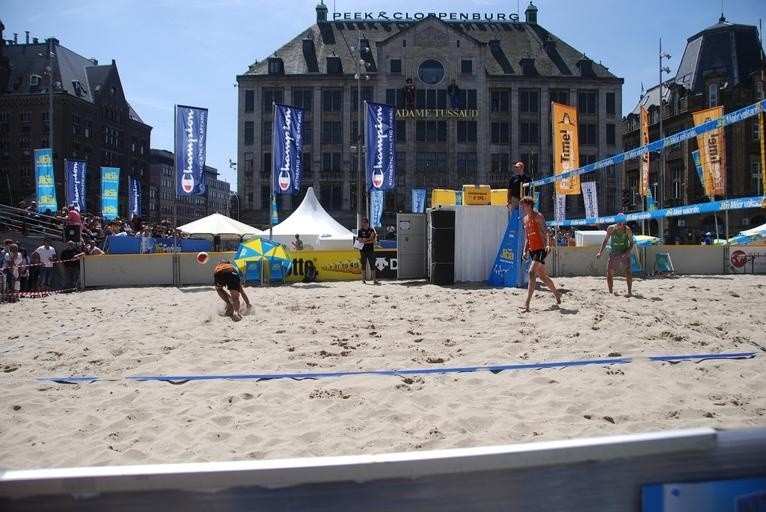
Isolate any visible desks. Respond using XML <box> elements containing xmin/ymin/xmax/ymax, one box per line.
<box><xmin>738</xmin><ymin>253</ymin><xmax>766</xmax><ymax>273</ymax></box>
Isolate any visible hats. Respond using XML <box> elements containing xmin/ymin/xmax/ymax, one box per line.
<box><xmin>515</xmin><ymin>162</ymin><xmax>526</xmax><ymax>171</ymax></box>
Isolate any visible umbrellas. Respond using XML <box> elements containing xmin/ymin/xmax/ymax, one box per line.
<box><xmin>176</xmin><ymin>213</ymin><xmax>262</xmax><ymax>251</ymax></box>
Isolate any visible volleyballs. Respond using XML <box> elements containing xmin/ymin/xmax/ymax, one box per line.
<box><xmin>197</xmin><ymin>251</ymin><xmax>208</xmax><ymax>264</ymax></box>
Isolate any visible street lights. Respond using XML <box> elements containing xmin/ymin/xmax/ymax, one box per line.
<box><xmin>346</xmin><ymin>32</ymin><xmax>370</xmax><ymax>239</ymax></box>
<box><xmin>657</xmin><ymin>36</ymin><xmax>672</xmax><ymax>246</ymax></box>
<box><xmin>37</xmin><ymin>38</ymin><xmax>63</xmax><ymax>152</ymax></box>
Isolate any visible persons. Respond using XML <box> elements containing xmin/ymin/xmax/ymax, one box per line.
<box><xmin>1</xmin><ymin>239</ymin><xmax>56</xmax><ymax>303</ymax></box>
<box><xmin>358</xmin><ymin>218</ymin><xmax>381</xmax><ymax>285</ymax></box>
<box><xmin>597</xmin><ymin>213</ymin><xmax>633</xmax><ymax>297</ymax></box>
<box><xmin>61</xmin><ymin>240</ymin><xmax>105</xmax><ymax>293</ymax></box>
<box><xmin>214</xmin><ymin>259</ymin><xmax>251</xmax><ymax>321</ymax></box>
<box><xmin>507</xmin><ymin>162</ymin><xmax>532</xmax><ymax>211</ymax></box>
<box><xmin>292</xmin><ymin>234</ymin><xmax>303</xmax><ymax>250</ymax></box>
<box><xmin>0</xmin><ymin>201</ymin><xmax>191</xmax><ymax>242</ymax></box>
<box><xmin>518</xmin><ymin>197</ymin><xmax>562</xmax><ymax>310</ymax></box>
<box><xmin>386</xmin><ymin>224</ymin><xmax>395</xmax><ymax>239</ymax></box>
